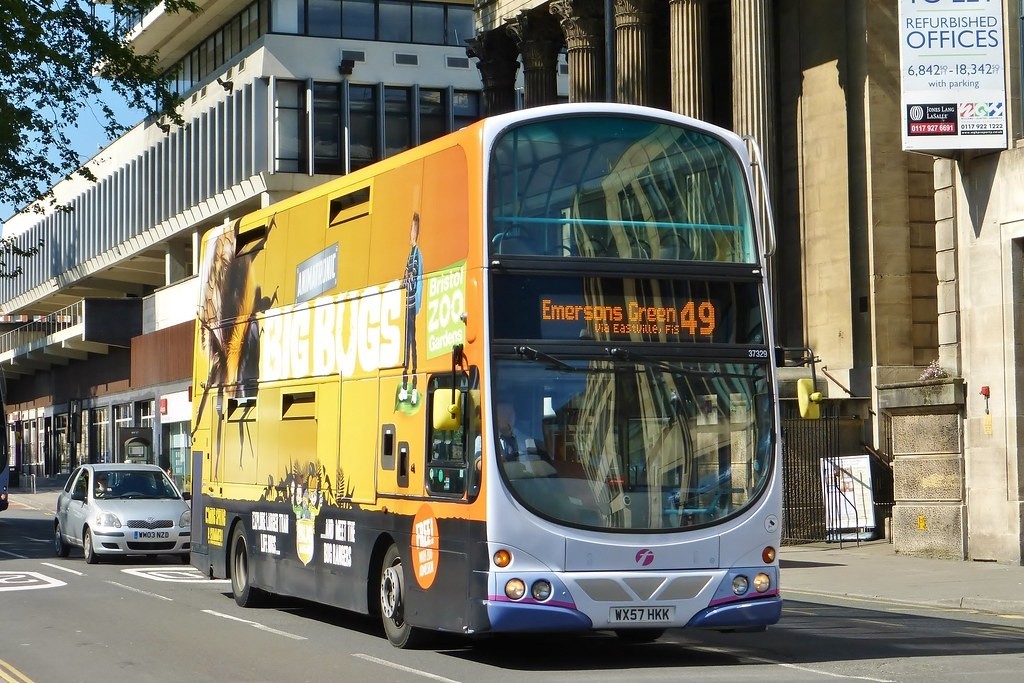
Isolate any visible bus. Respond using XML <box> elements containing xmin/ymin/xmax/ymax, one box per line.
<box><xmin>185</xmin><ymin>98</ymin><xmax>823</xmax><ymax>650</ymax></box>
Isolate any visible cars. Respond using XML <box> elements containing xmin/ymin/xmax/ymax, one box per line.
<box><xmin>52</xmin><ymin>463</ymin><xmax>193</xmax><ymax>564</ymax></box>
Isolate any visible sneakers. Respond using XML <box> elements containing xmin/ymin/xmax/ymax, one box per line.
<box><xmin>398</xmin><ymin>388</ymin><xmax>408</xmax><ymax>401</ymax></box>
<box><xmin>411</xmin><ymin>389</ymin><xmax>418</xmax><ymax>404</ymax></box>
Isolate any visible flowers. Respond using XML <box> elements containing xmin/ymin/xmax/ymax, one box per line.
<box><xmin>917</xmin><ymin>358</ymin><xmax>952</xmax><ymax>381</ymax></box>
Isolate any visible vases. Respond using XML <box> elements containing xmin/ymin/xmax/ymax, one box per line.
<box><xmin>875</xmin><ymin>377</ymin><xmax>964</xmax><ymax>409</ymax></box>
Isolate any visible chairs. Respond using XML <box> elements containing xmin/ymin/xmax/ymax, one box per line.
<box><xmin>659</xmin><ymin>234</ymin><xmax>695</xmax><ymax>260</ymax></box>
<box><xmin>492</xmin><ymin>231</ymin><xmax>540</xmax><ymax>255</ymax></box>
<box><xmin>548</xmin><ymin>246</ymin><xmax>577</xmax><ymax>255</ymax></box>
<box><xmin>605</xmin><ymin>228</ymin><xmax>653</xmax><ymax>258</ymax></box>
<box><xmin>571</xmin><ymin>238</ymin><xmax>608</xmax><ymax>257</ymax></box>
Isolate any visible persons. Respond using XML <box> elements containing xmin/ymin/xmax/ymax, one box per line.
<box><xmin>163</xmin><ymin>469</ymin><xmax>175</xmax><ymax>486</ymax></box>
<box><xmin>95</xmin><ymin>474</ymin><xmax>112</xmax><ymax>497</ymax></box>
<box><xmin>398</xmin><ymin>213</ymin><xmax>419</xmax><ymax>405</ymax></box>
<box><xmin>475</xmin><ymin>403</ymin><xmax>531</xmax><ymax>471</ymax></box>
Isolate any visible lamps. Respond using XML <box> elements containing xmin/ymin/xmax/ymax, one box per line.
<box><xmin>338</xmin><ymin>58</ymin><xmax>355</xmax><ymax>75</ymax></box>
<box><xmin>217</xmin><ymin>78</ymin><xmax>233</xmax><ymax>91</ymax></box>
